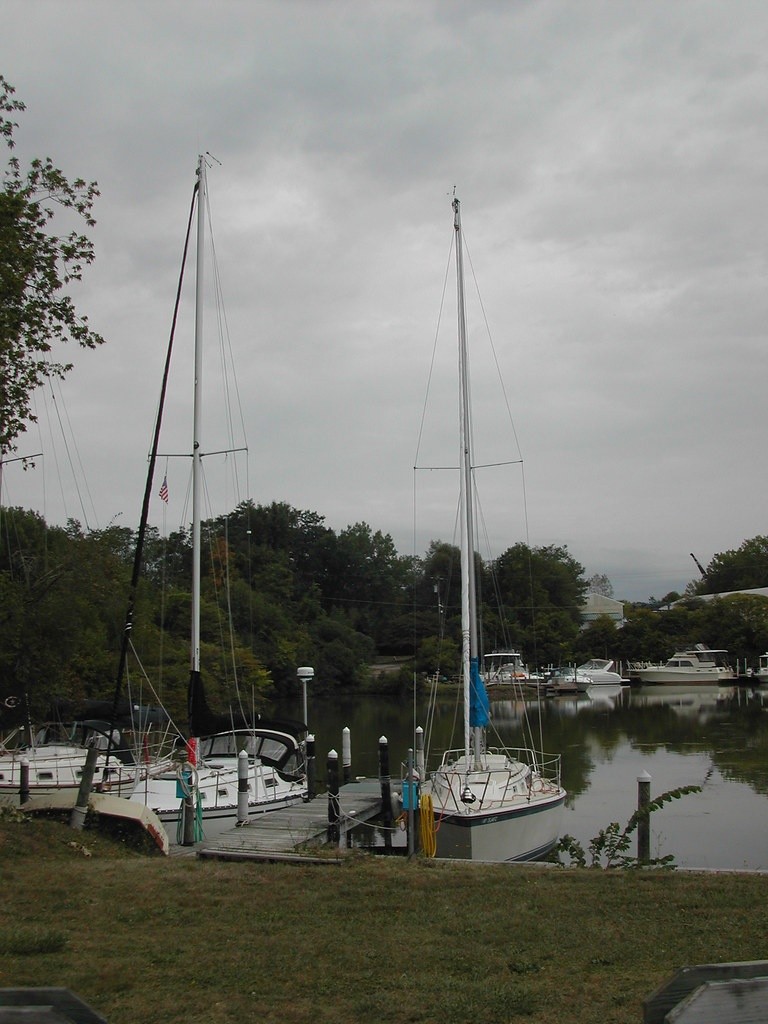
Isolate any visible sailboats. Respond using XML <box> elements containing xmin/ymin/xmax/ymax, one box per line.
<box><xmin>403</xmin><ymin>186</ymin><xmax>571</xmax><ymax>863</ymax></box>
<box><xmin>0</xmin><ymin>153</ymin><xmax>318</xmax><ymax>845</ymax></box>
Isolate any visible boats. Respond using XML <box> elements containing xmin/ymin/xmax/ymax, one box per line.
<box><xmin>479</xmin><ymin>642</ymin><xmax>768</xmax><ymax>692</ymax></box>
